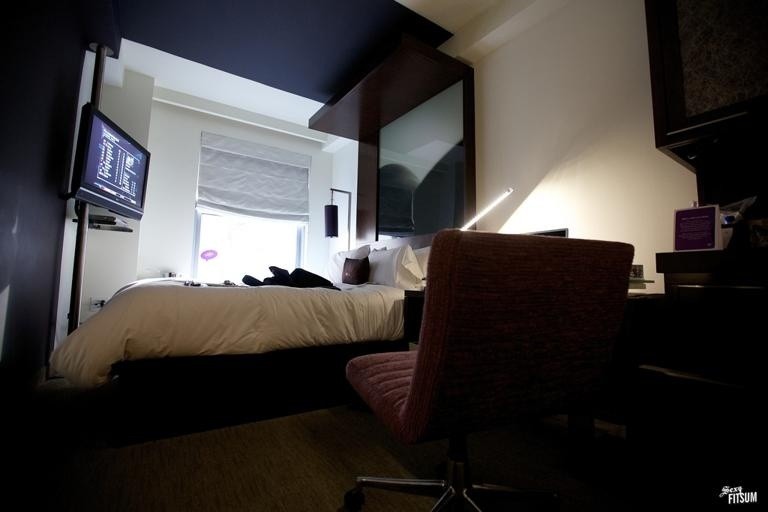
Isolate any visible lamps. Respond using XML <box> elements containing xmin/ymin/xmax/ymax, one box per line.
<box><xmin>323</xmin><ymin>188</ymin><xmax>351</xmax><ymax>252</ymax></box>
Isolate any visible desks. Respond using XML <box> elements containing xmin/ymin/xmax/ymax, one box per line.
<box><xmin>403</xmin><ymin>291</ymin><xmax>665</xmax><ymax>484</ymax></box>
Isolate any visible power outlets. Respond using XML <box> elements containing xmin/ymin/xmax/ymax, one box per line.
<box><xmin>90</xmin><ymin>297</ymin><xmax>107</xmax><ymax>312</ymax></box>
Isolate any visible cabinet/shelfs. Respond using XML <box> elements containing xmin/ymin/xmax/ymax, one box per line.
<box><xmin>638</xmin><ymin>0</ymin><xmax>767</xmax><ymax>512</ymax></box>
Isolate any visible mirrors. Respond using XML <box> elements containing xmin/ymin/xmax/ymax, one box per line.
<box><xmin>356</xmin><ymin>70</ymin><xmax>477</xmax><ymax>246</ymax></box>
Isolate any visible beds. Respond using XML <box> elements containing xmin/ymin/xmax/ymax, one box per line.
<box><xmin>46</xmin><ymin>242</ymin><xmax>432</xmax><ymax>444</ymax></box>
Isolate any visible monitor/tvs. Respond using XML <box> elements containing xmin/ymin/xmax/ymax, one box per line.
<box><xmin>72</xmin><ymin>101</ymin><xmax>151</xmax><ymax>222</ymax></box>
<box><xmin>523</xmin><ymin>228</ymin><xmax>568</xmax><ymax>238</ymax></box>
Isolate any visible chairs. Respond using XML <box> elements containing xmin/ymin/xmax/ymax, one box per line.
<box><xmin>344</xmin><ymin>229</ymin><xmax>634</xmax><ymax>511</ymax></box>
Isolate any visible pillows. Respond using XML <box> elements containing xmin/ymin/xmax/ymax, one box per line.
<box><xmin>342</xmin><ymin>256</ymin><xmax>369</xmax><ymax>285</ymax></box>
<box><xmin>367</xmin><ymin>245</ymin><xmax>424</xmax><ymax>289</ymax></box>
<box><xmin>322</xmin><ymin>246</ymin><xmax>369</xmax><ymax>284</ymax></box>
